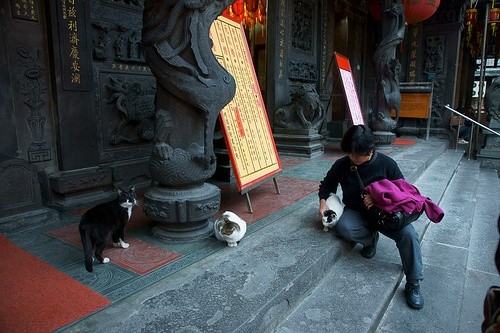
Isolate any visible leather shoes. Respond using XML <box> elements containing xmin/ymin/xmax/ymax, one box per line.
<box><xmin>405</xmin><ymin>282</ymin><xmax>424</xmax><ymax>308</ymax></box>
<box><xmin>361</xmin><ymin>233</ymin><xmax>380</xmax><ymax>258</ymax></box>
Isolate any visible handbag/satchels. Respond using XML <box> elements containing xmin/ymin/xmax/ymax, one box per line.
<box><xmin>350</xmin><ymin>160</ymin><xmax>424</xmax><ymax>230</ymax></box>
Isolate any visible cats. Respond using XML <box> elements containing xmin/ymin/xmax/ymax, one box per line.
<box><xmin>214</xmin><ymin>211</ymin><xmax>247</xmax><ymax>247</ymax></box>
<box><xmin>320</xmin><ymin>193</ymin><xmax>345</xmax><ymax>231</ymax></box>
<box><xmin>78</xmin><ymin>184</ymin><xmax>144</xmax><ymax>273</ymax></box>
